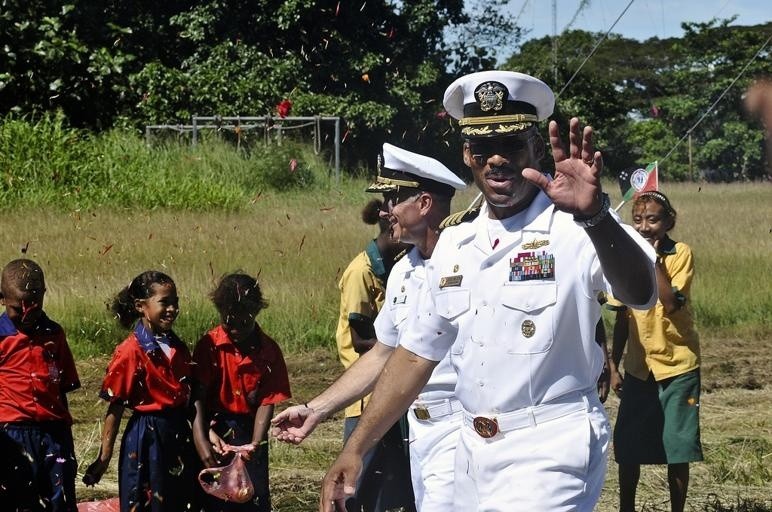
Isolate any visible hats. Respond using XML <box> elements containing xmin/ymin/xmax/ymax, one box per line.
<box><xmin>441</xmin><ymin>70</ymin><xmax>556</xmax><ymax>140</ymax></box>
<box><xmin>365</xmin><ymin>143</ymin><xmax>467</xmax><ymax>196</ymax></box>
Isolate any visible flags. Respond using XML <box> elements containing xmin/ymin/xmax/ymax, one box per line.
<box><xmin>615</xmin><ymin>160</ymin><xmax>657</xmax><ymax>200</ymax></box>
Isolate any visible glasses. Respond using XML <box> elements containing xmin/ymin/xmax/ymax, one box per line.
<box><xmin>466</xmin><ymin>134</ymin><xmax>536</xmax><ymax>157</ymax></box>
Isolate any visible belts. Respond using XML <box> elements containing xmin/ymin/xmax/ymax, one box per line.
<box><xmin>463</xmin><ymin>387</ymin><xmax>601</xmax><ymax>438</ymax></box>
<box><xmin>407</xmin><ymin>394</ymin><xmax>463</xmax><ymax>422</ymax></box>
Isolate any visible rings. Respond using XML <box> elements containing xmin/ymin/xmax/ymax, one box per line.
<box><xmin>331</xmin><ymin>500</ymin><xmax>336</xmax><ymax>504</ymax></box>
<box><xmin>579</xmin><ymin>153</ymin><xmax>595</xmax><ymax>164</ymax></box>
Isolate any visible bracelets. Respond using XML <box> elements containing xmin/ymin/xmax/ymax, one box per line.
<box><xmin>571</xmin><ymin>191</ymin><xmax>611</xmax><ymax>230</ymax></box>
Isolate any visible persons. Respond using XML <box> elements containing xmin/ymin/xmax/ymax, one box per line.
<box><xmin>319</xmin><ymin>71</ymin><xmax>656</xmax><ymax>511</ymax></box>
<box><xmin>608</xmin><ymin>186</ymin><xmax>705</xmax><ymax>511</ymax></box>
<box><xmin>79</xmin><ymin>271</ymin><xmax>231</xmax><ymax>511</ymax></box>
<box><xmin>187</xmin><ymin>269</ymin><xmax>292</xmax><ymax>512</ymax></box>
<box><xmin>592</xmin><ymin>289</ymin><xmax>611</xmax><ymax>404</ymax></box>
<box><xmin>330</xmin><ymin>196</ymin><xmax>416</xmax><ymax>511</ymax></box>
<box><xmin>2</xmin><ymin>258</ymin><xmax>85</xmax><ymax>511</ymax></box>
<box><xmin>267</xmin><ymin>141</ymin><xmax>469</xmax><ymax>512</ymax></box>
